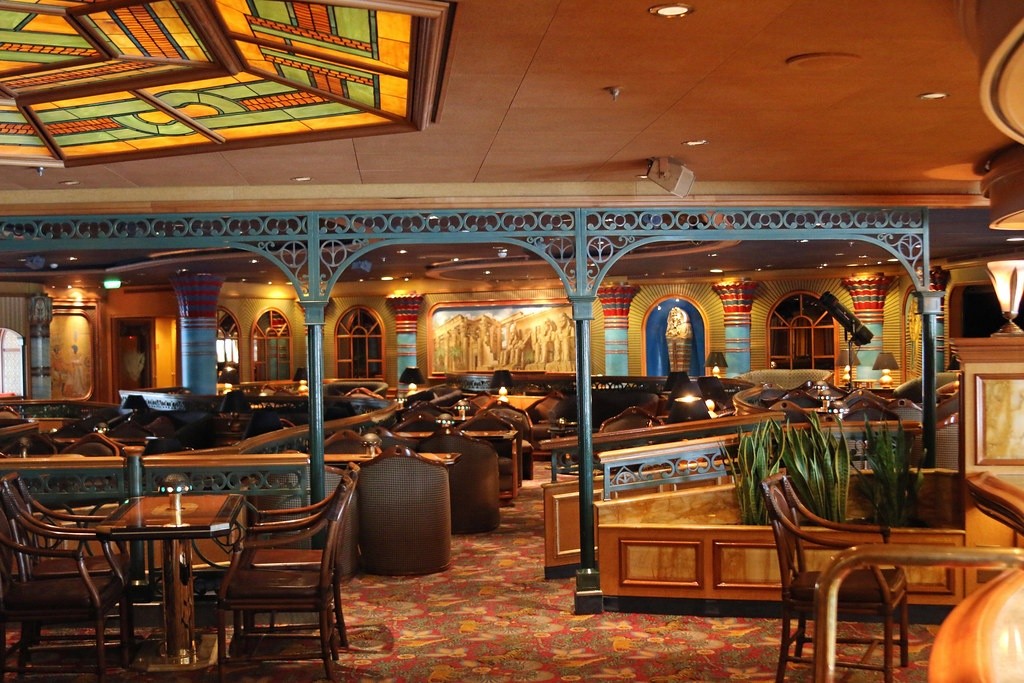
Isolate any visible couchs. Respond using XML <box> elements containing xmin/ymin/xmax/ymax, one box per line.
<box><xmin>0</xmin><ymin>369</ymin><xmax>962</xmax><ymax>576</ymax></box>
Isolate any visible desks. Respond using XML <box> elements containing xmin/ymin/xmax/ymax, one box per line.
<box><xmin>393</xmin><ymin>431</ymin><xmax>519</xmax><ymax>498</ymax></box>
<box><xmin>95</xmin><ymin>494</ymin><xmax>244</xmax><ymax>669</ymax></box>
<box><xmin>324</xmin><ymin>453</ymin><xmax>464</xmax><ymax>474</ymax></box>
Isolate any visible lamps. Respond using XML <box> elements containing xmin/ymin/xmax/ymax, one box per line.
<box><xmin>104</xmin><ymin>273</ymin><xmax>122</xmax><ymax>289</ymax></box>
<box><xmin>836</xmin><ymin>349</ymin><xmax>861</xmax><ymax>383</ymax></box>
<box><xmin>647</xmin><ymin>158</ymin><xmax>696</xmax><ymax>198</ymax></box>
<box><xmin>703</xmin><ymin>352</ymin><xmax>728</xmax><ymax>378</ymax></box>
<box><xmin>25</xmin><ymin>256</ymin><xmax>45</xmax><ymax>269</ymax></box>
<box><xmin>293</xmin><ymin>368</ymin><xmax>309</xmax><ymax>396</ymax></box>
<box><xmin>667</xmin><ymin>397</ymin><xmax>711</xmax><ymax>424</ymax></box>
<box><xmin>399</xmin><ymin>367</ymin><xmax>425</xmax><ymax>395</ymax></box>
<box><xmin>664</xmin><ymin>383</ymin><xmax>709</xmax><ymax>411</ymax></box>
<box><xmin>122</xmin><ymin>395</ymin><xmax>149</xmax><ymax>421</ymax></box>
<box><xmin>872</xmin><ymin>352</ymin><xmax>899</xmax><ymax>388</ymax></box>
<box><xmin>491</xmin><ymin>370</ymin><xmax>513</xmax><ymax>404</ymax></box>
<box><xmin>663</xmin><ymin>372</ymin><xmax>691</xmax><ymax>392</ymax></box>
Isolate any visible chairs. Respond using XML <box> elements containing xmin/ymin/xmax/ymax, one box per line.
<box><xmin>0</xmin><ymin>462</ymin><xmax>361</xmax><ymax>683</ymax></box>
<box><xmin>761</xmin><ymin>473</ymin><xmax>908</xmax><ymax>683</ymax></box>
